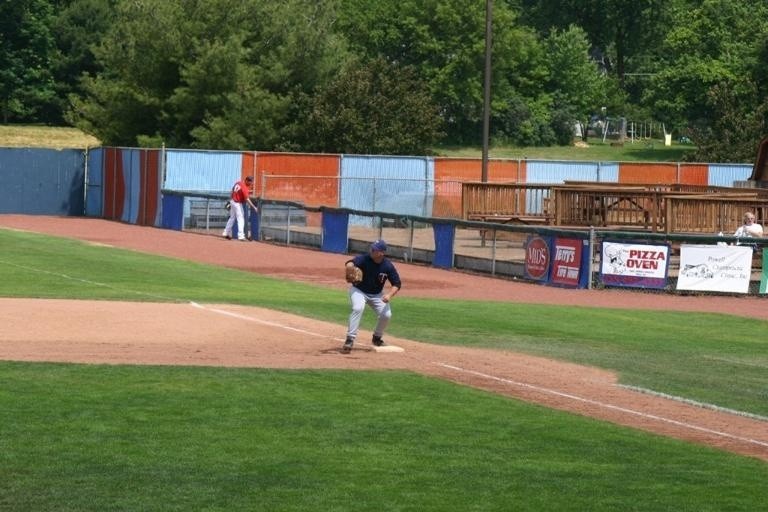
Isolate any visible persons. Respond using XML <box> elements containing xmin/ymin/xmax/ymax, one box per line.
<box><xmin>734</xmin><ymin>212</ymin><xmax>763</xmax><ymax>253</ymax></box>
<box><xmin>343</xmin><ymin>240</ymin><xmax>401</xmax><ymax>351</ymax></box>
<box><xmin>223</xmin><ymin>176</ymin><xmax>257</xmax><ymax>241</ymax></box>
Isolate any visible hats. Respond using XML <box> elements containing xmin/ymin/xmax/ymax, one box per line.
<box><xmin>246</xmin><ymin>176</ymin><xmax>254</xmax><ymax>183</ymax></box>
<box><xmin>370</xmin><ymin>239</ymin><xmax>387</xmax><ymax>251</ymax></box>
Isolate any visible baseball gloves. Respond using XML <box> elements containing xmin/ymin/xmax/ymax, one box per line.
<box><xmin>346</xmin><ymin>267</ymin><xmax>363</xmax><ymax>283</ymax></box>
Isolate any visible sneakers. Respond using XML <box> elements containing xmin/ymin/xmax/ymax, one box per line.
<box><xmin>372</xmin><ymin>339</ymin><xmax>385</xmax><ymax>346</ymax></box>
<box><xmin>224</xmin><ymin>235</ymin><xmax>231</xmax><ymax>240</ymax></box>
<box><xmin>342</xmin><ymin>339</ymin><xmax>352</xmax><ymax>350</ymax></box>
<box><xmin>239</xmin><ymin>238</ymin><xmax>250</xmax><ymax>242</ymax></box>
